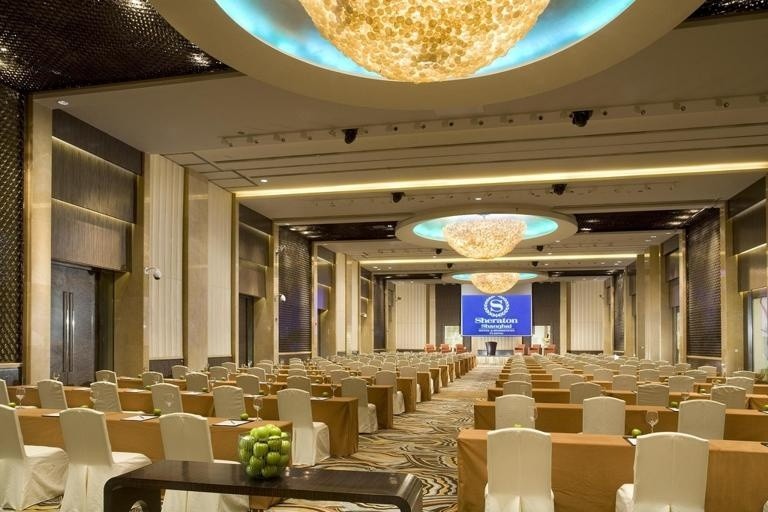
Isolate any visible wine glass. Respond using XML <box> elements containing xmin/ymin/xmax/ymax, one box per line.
<box><xmin>202</xmin><ymin>358</ymin><xmax>361</xmax><ymax>398</ymax></box>
<box><xmin>527</xmin><ymin>405</ymin><xmax>538</xmax><ymax>428</ymax></box>
<box><xmin>162</xmin><ymin>392</ymin><xmax>175</xmax><ymax>414</ymax></box>
<box><xmin>253</xmin><ymin>396</ymin><xmax>262</xmax><ymax>423</ymax></box>
<box><xmin>15</xmin><ymin>386</ymin><xmax>25</xmax><ymax>409</ymax></box>
<box><xmin>645</xmin><ymin>409</ymin><xmax>659</xmax><ymax>433</ymax></box>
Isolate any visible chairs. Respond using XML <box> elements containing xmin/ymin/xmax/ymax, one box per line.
<box><xmin>1</xmin><ymin>343</ymin><xmax>478</xmax><ymax>512</ymax></box>
<box><xmin>455</xmin><ymin>345</ymin><xmax>768</xmax><ymax>512</ymax></box>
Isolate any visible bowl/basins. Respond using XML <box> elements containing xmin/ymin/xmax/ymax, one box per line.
<box><xmin>237</xmin><ymin>432</ymin><xmax>290</xmax><ymax>479</ymax></box>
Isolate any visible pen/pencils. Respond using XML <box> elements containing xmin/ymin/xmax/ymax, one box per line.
<box><xmin>140</xmin><ymin>416</ymin><xmax>144</xmax><ymax>419</ymax></box>
<box><xmin>231</xmin><ymin>420</ymin><xmax>235</xmax><ymax>424</ymax></box>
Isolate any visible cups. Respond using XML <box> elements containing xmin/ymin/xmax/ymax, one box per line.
<box><xmin>681</xmin><ymin>391</ymin><xmax>690</xmax><ymax>400</ymax></box>
<box><xmin>101</xmin><ymin>373</ymin><xmax>109</xmax><ymax>381</ymax></box>
<box><xmin>152</xmin><ymin>376</ymin><xmax>159</xmax><ymax>384</ymax></box>
<box><xmin>53</xmin><ymin>371</ymin><xmax>60</xmax><ymax>381</ymax></box>
<box><xmin>89</xmin><ymin>390</ymin><xmax>97</xmax><ymax>411</ymax></box>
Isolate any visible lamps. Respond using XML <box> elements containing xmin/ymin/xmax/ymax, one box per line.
<box><xmin>144</xmin><ymin>265</ymin><xmax>161</xmax><ymax>280</ymax></box>
<box><xmin>440</xmin><ymin>217</ymin><xmax>527</xmax><ymax>261</ymax></box>
<box><xmin>471</xmin><ymin>272</ymin><xmax>521</xmax><ymax>295</ymax></box>
<box><xmin>298</xmin><ymin>1</ymin><xmax>553</xmax><ymax>84</ymax></box>
<box><xmin>275</xmin><ymin>294</ymin><xmax>286</xmax><ymax>303</ymax></box>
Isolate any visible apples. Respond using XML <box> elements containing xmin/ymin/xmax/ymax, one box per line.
<box><xmin>632</xmin><ymin>428</ymin><xmax>641</xmax><ymax>438</ymax></box>
<box><xmin>238</xmin><ymin>423</ymin><xmax>290</xmax><ymax>480</ymax></box>
<box><xmin>153</xmin><ymin>408</ymin><xmax>161</xmax><ymax>417</ymax></box>
<box><xmin>240</xmin><ymin>413</ymin><xmax>248</xmax><ymax>420</ymax></box>
<box><xmin>8</xmin><ymin>402</ymin><xmax>16</xmax><ymax>408</ymax></box>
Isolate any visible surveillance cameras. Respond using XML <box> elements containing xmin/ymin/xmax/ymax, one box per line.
<box><xmin>153</xmin><ymin>270</ymin><xmax>161</xmax><ymax>280</ymax></box>
<box><xmin>280</xmin><ymin>295</ymin><xmax>286</xmax><ymax>303</ymax></box>
